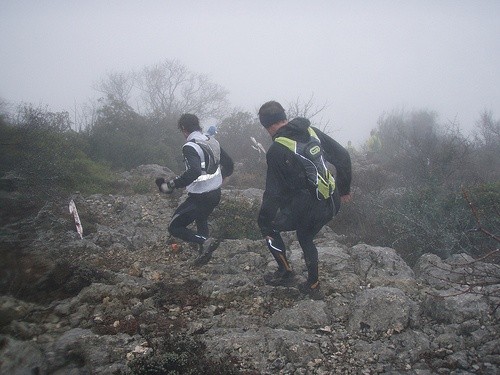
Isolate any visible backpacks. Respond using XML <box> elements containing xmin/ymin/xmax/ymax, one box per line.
<box><xmin>275</xmin><ymin>126</ymin><xmax>336</xmax><ymax>201</ymax></box>
<box><xmin>189</xmin><ymin>135</ymin><xmax>220</xmax><ymax>173</ymax></box>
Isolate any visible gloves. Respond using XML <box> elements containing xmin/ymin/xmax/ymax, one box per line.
<box><xmin>161</xmin><ymin>180</ymin><xmax>175</xmax><ymax>193</ymax></box>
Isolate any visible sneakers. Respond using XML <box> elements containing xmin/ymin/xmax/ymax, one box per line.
<box><xmin>193</xmin><ymin>237</ymin><xmax>219</xmax><ymax>265</ymax></box>
<box><xmin>299</xmin><ymin>281</ymin><xmax>326</xmax><ymax>300</ymax></box>
<box><xmin>264</xmin><ymin>269</ymin><xmax>296</xmax><ymax>288</ymax></box>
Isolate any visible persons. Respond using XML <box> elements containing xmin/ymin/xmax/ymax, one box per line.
<box><xmin>258</xmin><ymin>100</ymin><xmax>352</xmax><ymax>296</ymax></box>
<box><xmin>156</xmin><ymin>113</ymin><xmax>234</xmax><ymax>266</ymax></box>
